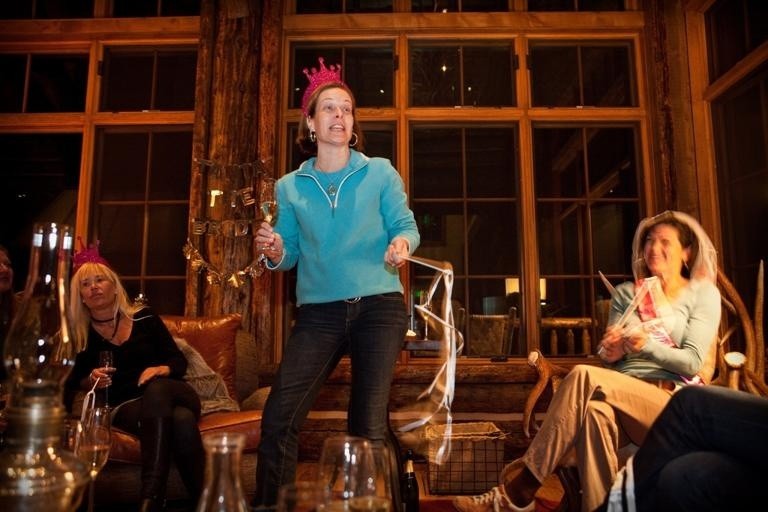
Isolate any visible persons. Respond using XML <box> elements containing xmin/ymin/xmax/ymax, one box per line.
<box><xmin>62</xmin><ymin>262</ymin><xmax>203</xmax><ymax>512</ymax></box>
<box><xmin>452</xmin><ymin>211</ymin><xmax>721</xmax><ymax>512</ymax></box>
<box><xmin>0</xmin><ymin>244</ymin><xmax>15</xmax><ymax>381</ymax></box>
<box><xmin>587</xmin><ymin>384</ymin><xmax>768</xmax><ymax>511</ymax></box>
<box><xmin>253</xmin><ymin>79</ymin><xmax>422</xmax><ymax>512</ymax></box>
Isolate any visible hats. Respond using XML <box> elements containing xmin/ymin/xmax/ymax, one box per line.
<box><xmin>68</xmin><ymin>235</ymin><xmax>113</xmax><ymax>277</ymax></box>
<box><xmin>300</xmin><ymin>54</ymin><xmax>350</xmax><ymax>118</ymax></box>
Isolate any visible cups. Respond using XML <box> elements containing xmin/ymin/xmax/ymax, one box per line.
<box><xmin>314</xmin><ymin>437</ymin><xmax>399</xmax><ymax>511</ymax></box>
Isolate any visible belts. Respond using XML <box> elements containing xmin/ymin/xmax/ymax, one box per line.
<box><xmin>332</xmin><ymin>290</ymin><xmax>404</xmax><ymax>304</ymax></box>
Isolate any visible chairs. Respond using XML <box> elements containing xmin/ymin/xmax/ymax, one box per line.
<box><xmin>523</xmin><ymin>272</ymin><xmax>768</xmax><ymax>512</ymax></box>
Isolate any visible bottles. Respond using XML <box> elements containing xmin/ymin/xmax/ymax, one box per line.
<box><xmin>0</xmin><ymin>220</ymin><xmax>88</xmax><ymax>510</ymax></box>
<box><xmin>198</xmin><ymin>433</ymin><xmax>251</xmax><ymax>511</ymax></box>
<box><xmin>399</xmin><ymin>448</ymin><xmax>419</xmax><ymax>511</ymax></box>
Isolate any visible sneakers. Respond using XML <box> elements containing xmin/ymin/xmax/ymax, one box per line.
<box><xmin>451</xmin><ymin>482</ymin><xmax>536</xmax><ymax>512</ymax></box>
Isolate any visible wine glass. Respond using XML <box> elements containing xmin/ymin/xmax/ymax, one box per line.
<box><xmin>418</xmin><ymin>290</ymin><xmax>433</xmax><ymax>341</ymax></box>
<box><xmin>98</xmin><ymin>349</ymin><xmax>114</xmax><ymax>416</ymax></box>
<box><xmin>255</xmin><ymin>178</ymin><xmax>280</xmax><ymax>253</ymax></box>
<box><xmin>76</xmin><ymin>406</ymin><xmax>112</xmax><ymax>511</ymax></box>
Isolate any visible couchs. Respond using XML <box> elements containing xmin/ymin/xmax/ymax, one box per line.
<box><xmin>64</xmin><ymin>311</ymin><xmax>279</xmax><ymax>512</ymax></box>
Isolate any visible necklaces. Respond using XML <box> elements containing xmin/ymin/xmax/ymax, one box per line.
<box><xmin>315</xmin><ymin>157</ymin><xmax>351</xmax><ymax>196</ymax></box>
<box><xmin>89</xmin><ymin>314</ymin><xmax>121</xmax><ymax>342</ymax></box>
